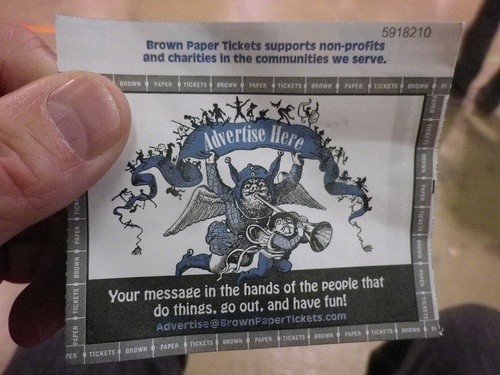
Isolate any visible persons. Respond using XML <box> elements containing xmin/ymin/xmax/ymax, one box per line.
<box><xmin>0</xmin><ymin>15</ymin><xmax>500</xmax><ymax>375</ymax></box>
<box><xmin>447</xmin><ymin>0</ymin><xmax>500</xmax><ymax>115</ymax></box>
